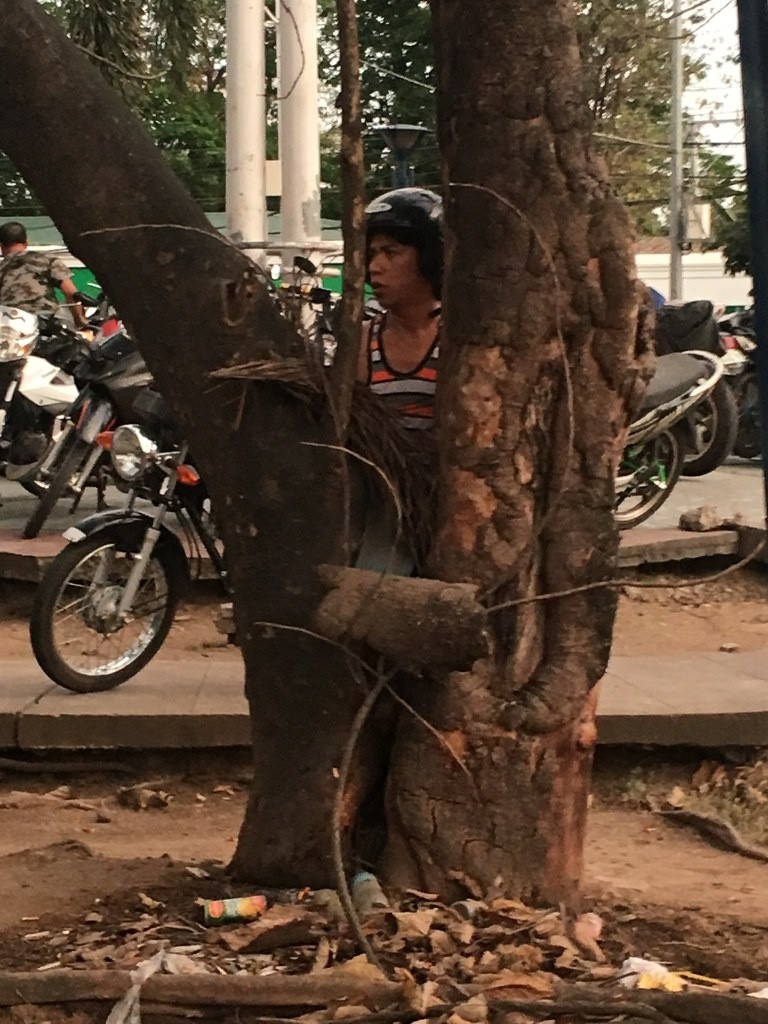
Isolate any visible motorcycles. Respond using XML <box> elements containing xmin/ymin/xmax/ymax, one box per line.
<box><xmin>0</xmin><ymin>255</ymin><xmax>767</xmax><ymax>695</ymax></box>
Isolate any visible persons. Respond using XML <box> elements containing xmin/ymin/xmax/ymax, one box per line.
<box><xmin>357</xmin><ymin>186</ymin><xmax>443</xmax><ymax>456</ymax></box>
<box><xmin>0</xmin><ymin>221</ymin><xmax>88</xmax><ymax>331</ymax></box>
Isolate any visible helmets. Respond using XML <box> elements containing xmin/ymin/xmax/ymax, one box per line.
<box><xmin>365</xmin><ymin>187</ymin><xmax>443</xmax><ymax>238</ymax></box>
